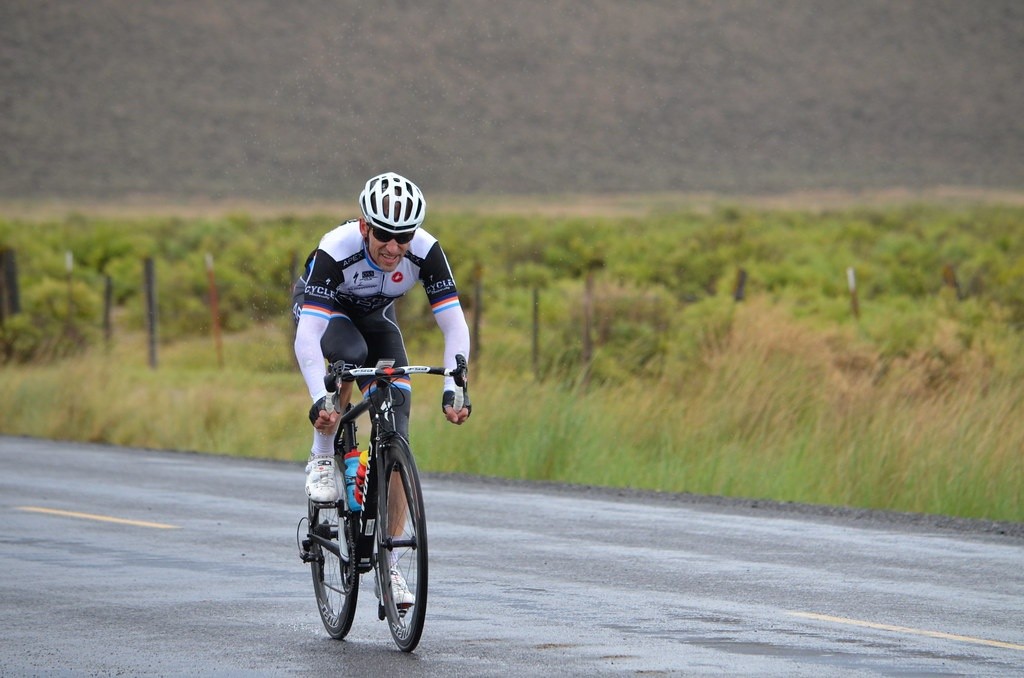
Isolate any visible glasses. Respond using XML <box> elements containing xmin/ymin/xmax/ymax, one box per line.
<box><xmin>365</xmin><ymin>220</ymin><xmax>416</xmax><ymax>244</ymax></box>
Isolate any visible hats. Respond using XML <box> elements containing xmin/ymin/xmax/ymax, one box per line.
<box><xmin>360</xmin><ymin>171</ymin><xmax>427</xmax><ymax>232</ymax></box>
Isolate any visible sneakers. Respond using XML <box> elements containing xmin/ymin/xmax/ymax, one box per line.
<box><xmin>305</xmin><ymin>454</ymin><xmax>339</xmax><ymax>504</ymax></box>
<box><xmin>374</xmin><ymin>570</ymin><xmax>416</xmax><ymax>608</ymax></box>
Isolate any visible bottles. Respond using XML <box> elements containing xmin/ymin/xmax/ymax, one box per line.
<box><xmin>345</xmin><ymin>449</ymin><xmax>363</xmax><ymax>512</ymax></box>
<box><xmin>354</xmin><ymin>450</ymin><xmax>369</xmax><ymax>505</ymax></box>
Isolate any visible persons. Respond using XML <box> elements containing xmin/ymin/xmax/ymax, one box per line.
<box><xmin>292</xmin><ymin>171</ymin><xmax>471</xmax><ymax>609</ymax></box>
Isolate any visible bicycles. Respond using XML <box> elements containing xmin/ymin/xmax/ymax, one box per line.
<box><xmin>297</xmin><ymin>322</ymin><xmax>470</xmax><ymax>652</ymax></box>
